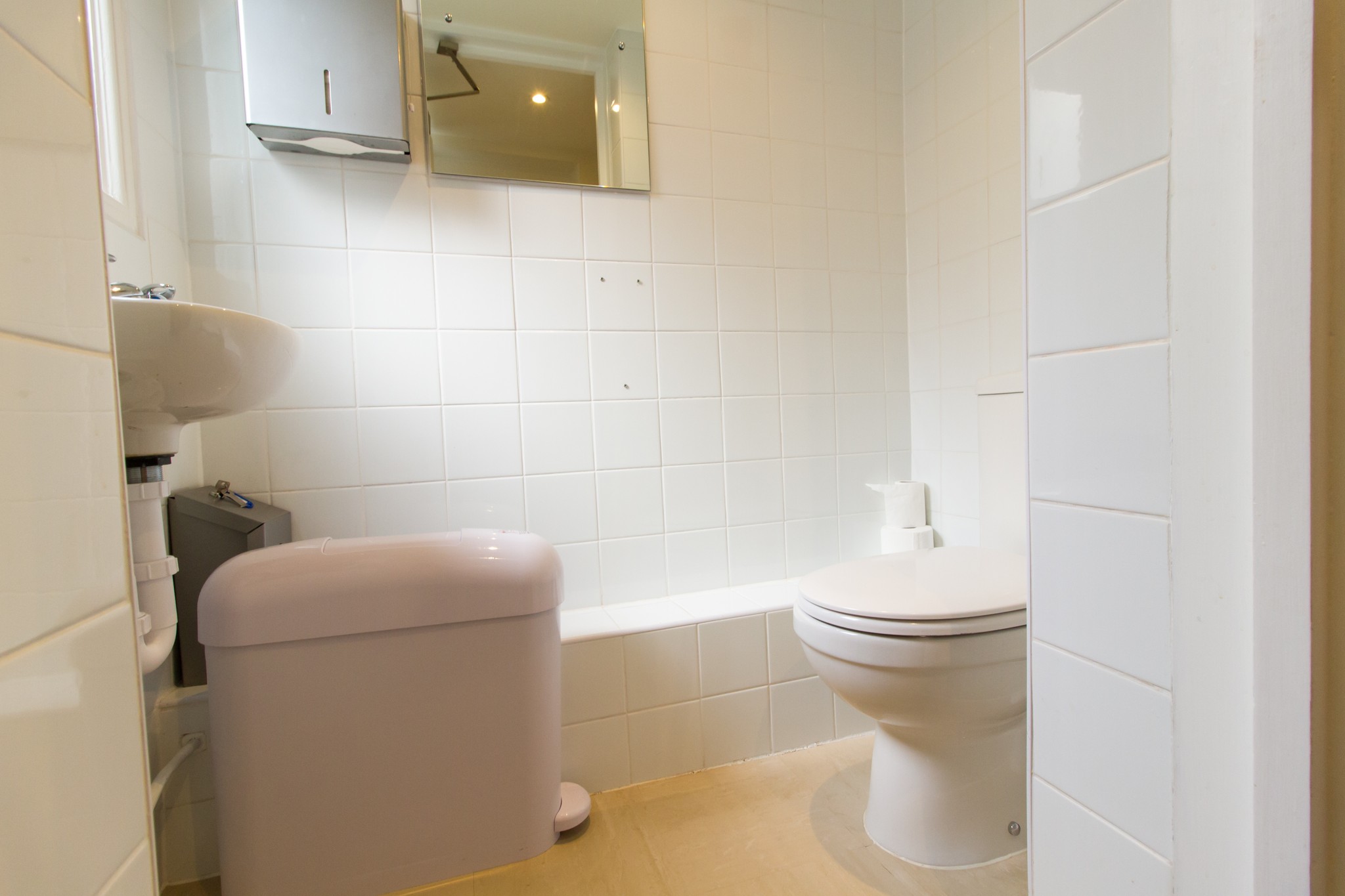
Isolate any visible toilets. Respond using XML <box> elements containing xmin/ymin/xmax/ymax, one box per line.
<box><xmin>789</xmin><ymin>388</ymin><xmax>1028</xmax><ymax>874</ymax></box>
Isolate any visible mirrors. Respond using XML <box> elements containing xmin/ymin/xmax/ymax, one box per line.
<box><xmin>415</xmin><ymin>0</ymin><xmax>652</xmax><ymax>193</ymax></box>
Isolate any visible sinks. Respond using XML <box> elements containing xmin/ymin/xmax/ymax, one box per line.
<box><xmin>112</xmin><ymin>296</ymin><xmax>299</xmax><ymax>425</ymax></box>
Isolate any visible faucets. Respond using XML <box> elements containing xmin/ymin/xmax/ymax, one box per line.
<box><xmin>109</xmin><ymin>253</ymin><xmax>144</xmax><ymax>298</ymax></box>
<box><xmin>139</xmin><ymin>281</ymin><xmax>176</xmax><ymax>300</ymax></box>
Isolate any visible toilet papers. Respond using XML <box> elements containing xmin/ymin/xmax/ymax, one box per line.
<box><xmin>866</xmin><ymin>480</ymin><xmax>935</xmax><ymax>552</ymax></box>
